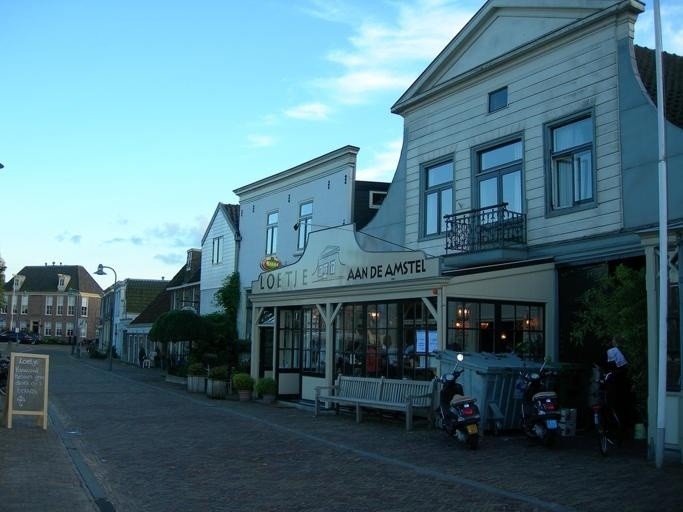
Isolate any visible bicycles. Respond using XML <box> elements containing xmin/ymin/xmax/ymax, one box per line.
<box><xmin>590</xmin><ymin>363</ymin><xmax>610</xmax><ymax>456</ymax></box>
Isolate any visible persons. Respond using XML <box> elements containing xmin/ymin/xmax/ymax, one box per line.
<box><xmin>355</xmin><ymin>324</ymin><xmax>420</xmax><ymax>377</ymax></box>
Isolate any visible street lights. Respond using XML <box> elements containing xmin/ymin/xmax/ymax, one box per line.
<box><xmin>93</xmin><ymin>264</ymin><xmax>117</xmax><ymax>359</ymax></box>
<box><xmin>67</xmin><ymin>288</ymin><xmax>82</xmax><ymax>353</ymax></box>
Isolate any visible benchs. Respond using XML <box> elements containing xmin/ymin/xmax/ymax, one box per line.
<box><xmin>312</xmin><ymin>373</ymin><xmax>437</xmax><ymax>432</ymax></box>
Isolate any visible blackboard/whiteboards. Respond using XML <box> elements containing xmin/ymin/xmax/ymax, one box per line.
<box><xmin>8</xmin><ymin>352</ymin><xmax>50</xmax><ymax>415</ymax></box>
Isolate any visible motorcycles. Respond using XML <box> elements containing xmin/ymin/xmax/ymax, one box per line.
<box><xmin>515</xmin><ymin>356</ymin><xmax>562</xmax><ymax>449</ymax></box>
<box><xmin>430</xmin><ymin>353</ymin><xmax>481</xmax><ymax>451</ymax></box>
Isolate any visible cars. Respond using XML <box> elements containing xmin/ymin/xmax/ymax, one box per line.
<box><xmin>0</xmin><ymin>331</ymin><xmax>33</xmax><ymax>344</ymax></box>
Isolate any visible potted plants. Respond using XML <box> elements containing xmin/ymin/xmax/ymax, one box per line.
<box><xmin>186</xmin><ymin>352</ymin><xmax>276</xmax><ymax>405</ymax></box>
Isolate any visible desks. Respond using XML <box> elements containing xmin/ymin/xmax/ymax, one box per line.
<box><xmin>143</xmin><ymin>359</ymin><xmax>150</xmax><ymax>368</ymax></box>
